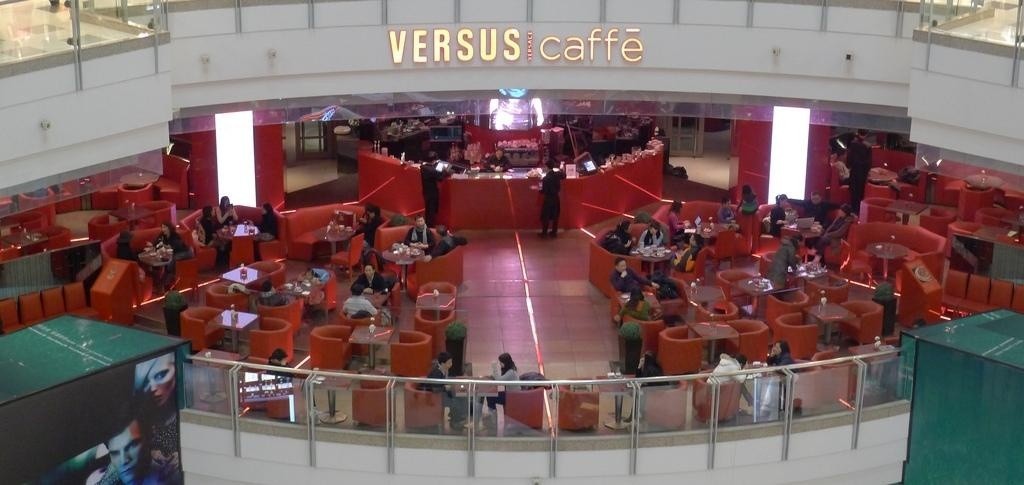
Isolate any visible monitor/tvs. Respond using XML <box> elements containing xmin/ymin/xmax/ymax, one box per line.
<box><xmin>435</xmin><ymin>161</ymin><xmax>452</xmax><ymax>173</ymax></box>
<box><xmin>582</xmin><ymin>160</ymin><xmax>597</xmax><ymax>174</ymax></box>
<box><xmin>488</xmin><ymin>98</ymin><xmax>531</xmax><ymax>131</ymax></box>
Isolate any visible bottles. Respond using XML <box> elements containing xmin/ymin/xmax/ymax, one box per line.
<box><xmin>639</xmin><ymin>240</ymin><xmax>644</xmax><ymax>250</ymax></box>
<box><xmin>239</xmin><ymin>262</ymin><xmax>247</xmax><ymax>277</ymax></box>
<box><xmin>820</xmin><ymin>298</ymin><xmax>826</xmax><ymax>308</ymax></box>
<box><xmin>381</xmin><ymin>148</ymin><xmax>388</xmax><ymax>158</ymax></box>
<box><xmin>368</xmin><ymin>318</ymin><xmax>376</xmax><ymax>336</ymax></box>
<box><xmin>325</xmin><ymin>222</ymin><xmax>351</xmax><ymax>234</ymax></box>
<box><xmin>244</xmin><ymin>221</ymin><xmax>255</xmax><ymax>233</ymax></box>
<box><xmin>697</xmin><ymin>225</ymin><xmax>702</xmax><ymax>235</ymax></box>
<box><xmin>230</xmin><ymin>303</ymin><xmax>239</xmax><ymax>321</ymax></box>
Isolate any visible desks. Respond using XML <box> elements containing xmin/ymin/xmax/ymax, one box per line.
<box><xmin>454</xmin><ymin>374</ymin><xmax>497</xmax><ymax>422</ymax></box>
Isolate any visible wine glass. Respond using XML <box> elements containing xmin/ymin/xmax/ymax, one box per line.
<box><xmin>602</xmin><ymin>152</ymin><xmax>634</xmax><ymax>166</ymax></box>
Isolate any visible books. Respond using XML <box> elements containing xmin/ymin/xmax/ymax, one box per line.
<box><xmin>435</xmin><ymin>161</ymin><xmax>449</xmax><ymax>174</ymax></box>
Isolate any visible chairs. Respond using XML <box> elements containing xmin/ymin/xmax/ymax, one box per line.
<box><xmin>589</xmin><ymin>189</ymin><xmax>907</xmax><ymax>436</ymax></box>
<box><xmin>180</xmin><ymin>202</ymin><xmax>466</xmax><ymax>431</ymax></box>
<box><xmin>1</xmin><ymin>154</ymin><xmax>218</xmax><ymax>334</ymax></box>
<box><xmin>502</xmin><ymin>371</ymin><xmax>598</xmax><ymax>433</ymax></box>
<box><xmin>830</xmin><ymin>145</ymin><xmax>1023</xmax><ymax>316</ymax></box>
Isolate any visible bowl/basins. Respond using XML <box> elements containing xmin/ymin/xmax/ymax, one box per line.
<box><xmin>283</xmin><ymin>279</ymin><xmax>313</xmax><ymax>294</ymax></box>
<box><xmin>393</xmin><ymin>243</ymin><xmax>419</xmax><ymax>257</ymax></box>
<box><xmin>636</xmin><ymin>244</ymin><xmax>669</xmax><ymax>258</ymax></box>
<box><xmin>753</xmin><ymin>278</ymin><xmax>769</xmax><ymax>289</ymax></box>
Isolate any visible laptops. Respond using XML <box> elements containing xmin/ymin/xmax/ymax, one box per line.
<box><xmin>792</xmin><ymin>218</ymin><xmax>814</xmax><ymax>229</ymax></box>
<box><xmin>688</xmin><ymin>216</ymin><xmax>702</xmax><ymax>229</ymax></box>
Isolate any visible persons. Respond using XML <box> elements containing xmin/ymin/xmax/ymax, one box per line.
<box><xmin>482</xmin><ymin>146</ymin><xmax>514</xmax><ymax>173</ymax></box>
<box><xmin>83</xmin><ymin>412</ymin><xmax>167</xmax><ymax>485</ymax></box>
<box><xmin>827</xmin><ymin>150</ymin><xmax>850</xmax><ymax>187</ymax></box>
<box><xmin>124</xmin><ymin>351</ymin><xmax>185</xmax><ymax>485</ymax></box>
<box><xmin>418</xmin><ymin>150</ymin><xmax>453</xmax><ymax>227</ymax></box>
<box><xmin>535</xmin><ymin>159</ymin><xmax>568</xmax><ymax>240</ymax></box>
<box><xmin>481</xmin><ymin>352</ymin><xmax>521</xmax><ymax>411</ymax></box>
<box><xmin>848</xmin><ymin>130</ymin><xmax>873</xmax><ymax>216</ymax></box>
<box><xmin>417</xmin><ymin>348</ymin><xmax>468</xmax><ymax>433</ymax></box>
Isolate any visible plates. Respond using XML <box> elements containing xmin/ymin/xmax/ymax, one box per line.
<box><xmin>526</xmin><ymin>173</ymin><xmax>540</xmax><ymax>177</ymax></box>
